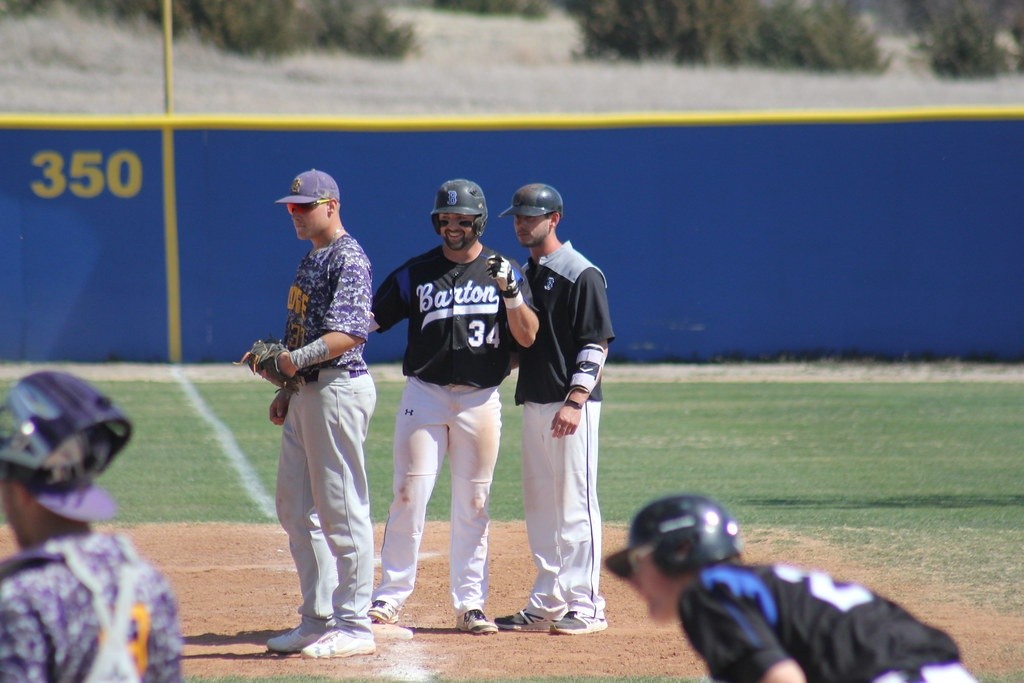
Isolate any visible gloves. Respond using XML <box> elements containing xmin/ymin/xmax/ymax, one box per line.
<box><xmin>485</xmin><ymin>254</ymin><xmax>524</xmax><ymax>298</ymax></box>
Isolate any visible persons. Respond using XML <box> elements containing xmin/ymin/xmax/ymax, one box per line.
<box><xmin>603</xmin><ymin>493</ymin><xmax>977</xmax><ymax>683</ymax></box>
<box><xmin>498</xmin><ymin>184</ymin><xmax>616</xmax><ymax>634</ymax></box>
<box><xmin>368</xmin><ymin>179</ymin><xmax>540</xmax><ymax>636</ymax></box>
<box><xmin>0</xmin><ymin>368</ymin><xmax>181</xmax><ymax>683</ymax></box>
<box><xmin>234</xmin><ymin>169</ymin><xmax>376</xmax><ymax>659</ymax></box>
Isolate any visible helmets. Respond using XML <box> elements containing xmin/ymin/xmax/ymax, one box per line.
<box><xmin>605</xmin><ymin>493</ymin><xmax>742</xmax><ymax>577</ymax></box>
<box><xmin>430</xmin><ymin>179</ymin><xmax>487</xmax><ymax>237</ymax></box>
<box><xmin>499</xmin><ymin>184</ymin><xmax>563</xmax><ymax>219</ymax></box>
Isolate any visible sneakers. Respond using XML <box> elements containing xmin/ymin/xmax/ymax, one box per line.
<box><xmin>267</xmin><ymin>626</ymin><xmax>325</xmax><ymax>652</ymax></box>
<box><xmin>494</xmin><ymin>608</ymin><xmax>559</xmax><ymax>632</ymax></box>
<box><xmin>456</xmin><ymin>609</ymin><xmax>498</xmax><ymax>635</ymax></box>
<box><xmin>300</xmin><ymin>628</ymin><xmax>375</xmax><ymax>658</ymax></box>
<box><xmin>550</xmin><ymin>610</ymin><xmax>608</xmax><ymax>634</ymax></box>
<box><xmin>367</xmin><ymin>600</ymin><xmax>399</xmax><ymax>624</ymax></box>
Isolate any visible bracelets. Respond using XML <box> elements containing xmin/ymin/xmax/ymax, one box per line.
<box><xmin>564</xmin><ymin>399</ymin><xmax>582</xmax><ymax>409</ymax></box>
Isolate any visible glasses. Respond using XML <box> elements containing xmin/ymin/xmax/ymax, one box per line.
<box><xmin>287</xmin><ymin>199</ymin><xmax>329</xmax><ymax>216</ymax></box>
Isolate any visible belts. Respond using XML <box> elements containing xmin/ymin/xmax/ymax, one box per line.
<box><xmin>896</xmin><ymin>668</ymin><xmax>926</xmax><ymax>683</ymax></box>
<box><xmin>294</xmin><ymin>369</ymin><xmax>368</xmax><ymax>385</ymax></box>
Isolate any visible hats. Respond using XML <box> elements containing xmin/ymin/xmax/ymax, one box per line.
<box><xmin>274</xmin><ymin>168</ymin><xmax>339</xmax><ymax>203</ymax></box>
<box><xmin>25</xmin><ymin>430</ymin><xmax>117</xmax><ymax>522</ymax></box>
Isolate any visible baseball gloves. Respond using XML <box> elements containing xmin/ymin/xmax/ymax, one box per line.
<box><xmin>247</xmin><ymin>338</ymin><xmax>301</xmax><ymax>394</ymax></box>
